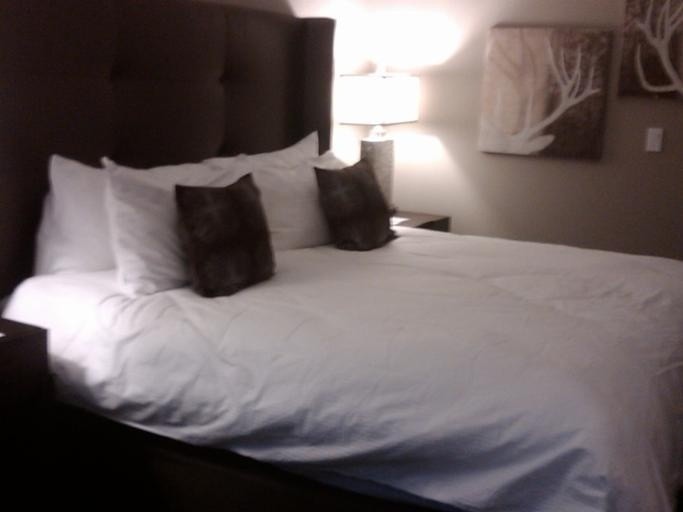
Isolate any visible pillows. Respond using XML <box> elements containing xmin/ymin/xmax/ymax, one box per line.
<box><xmin>40</xmin><ymin>133</ymin><xmax>349</xmax><ymax>299</ymax></box>
<box><xmin>313</xmin><ymin>158</ymin><xmax>398</xmax><ymax>252</ymax></box>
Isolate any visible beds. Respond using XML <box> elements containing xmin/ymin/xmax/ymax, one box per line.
<box><xmin>2</xmin><ymin>8</ymin><xmax>683</xmax><ymax>512</ymax></box>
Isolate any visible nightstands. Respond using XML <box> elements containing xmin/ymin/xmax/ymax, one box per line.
<box><xmin>387</xmin><ymin>211</ymin><xmax>450</xmax><ymax>234</ymax></box>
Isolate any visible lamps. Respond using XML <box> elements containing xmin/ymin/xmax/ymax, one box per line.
<box><xmin>337</xmin><ymin>72</ymin><xmax>425</xmax><ymax>210</ymax></box>
